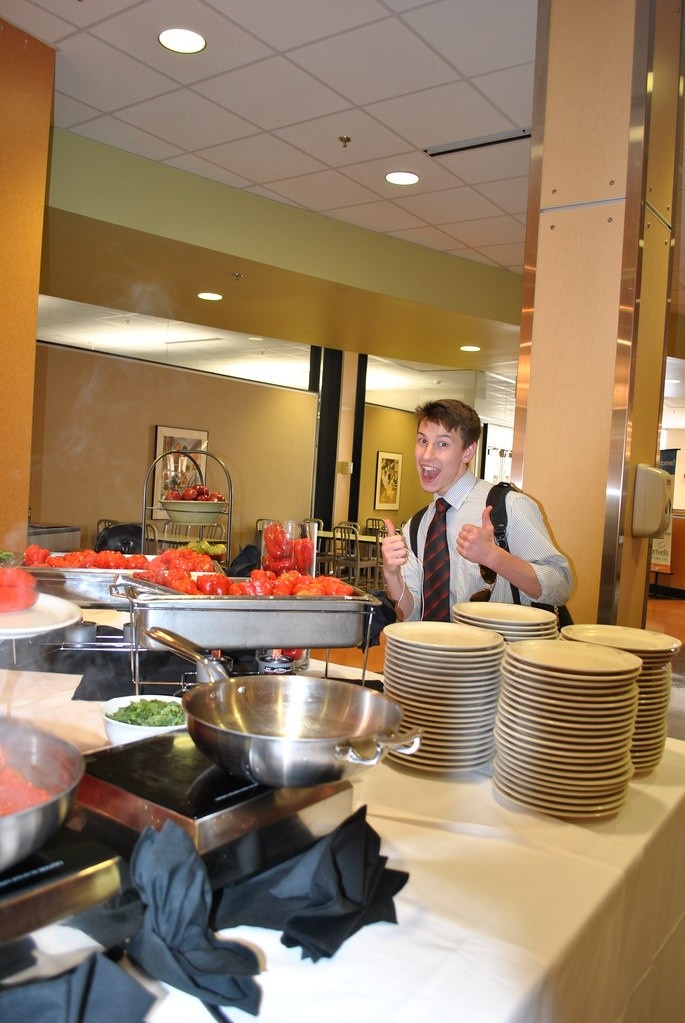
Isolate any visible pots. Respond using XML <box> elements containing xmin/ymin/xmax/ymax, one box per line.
<box><xmin>142</xmin><ymin>626</ymin><xmax>404</xmax><ymax>787</ymax></box>
<box><xmin>0</xmin><ymin>719</ymin><xmax>85</xmax><ymax>873</ymax></box>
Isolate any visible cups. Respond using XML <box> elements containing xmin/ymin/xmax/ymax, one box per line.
<box><xmin>256</xmin><ymin>518</ymin><xmax>318</xmax><ymax>672</ymax></box>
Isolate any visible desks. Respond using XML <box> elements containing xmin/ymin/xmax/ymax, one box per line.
<box><xmin>143</xmin><ymin>531</ymin><xmax>227</xmax><ymax>557</ymax></box>
<box><xmin>365</xmin><ymin>526</ymin><xmax>400</xmax><ymax>538</ymax></box>
<box><xmin>116</xmin><ymin>657</ymin><xmax>683</xmax><ymax>1023</ymax></box>
<box><xmin>314</xmin><ymin>530</ymin><xmax>384</xmax><ymax>591</ymax></box>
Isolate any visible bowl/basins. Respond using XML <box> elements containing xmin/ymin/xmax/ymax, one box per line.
<box><xmin>158</xmin><ymin>500</ymin><xmax>230</xmax><ymax>524</ymax></box>
<box><xmin>99</xmin><ymin>695</ymin><xmax>188</xmax><ymax>746</ymax></box>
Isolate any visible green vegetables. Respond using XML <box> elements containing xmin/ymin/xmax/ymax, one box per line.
<box><xmin>104</xmin><ymin>698</ymin><xmax>186</xmax><ymax>727</ymax></box>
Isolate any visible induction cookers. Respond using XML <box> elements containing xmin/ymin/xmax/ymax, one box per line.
<box><xmin>0</xmin><ymin>731</ymin><xmax>353</xmax><ymax>995</ymax></box>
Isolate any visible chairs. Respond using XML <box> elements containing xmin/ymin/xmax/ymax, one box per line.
<box><xmin>97</xmin><ymin>518</ymin><xmax>225</xmax><ymax>556</ymax></box>
<box><xmin>254</xmin><ymin>517</ymin><xmax>410</xmax><ymax>592</ymax></box>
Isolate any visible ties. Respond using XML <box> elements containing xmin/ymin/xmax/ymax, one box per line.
<box><xmin>420</xmin><ymin>497</ymin><xmax>452</xmax><ymax>622</ymax></box>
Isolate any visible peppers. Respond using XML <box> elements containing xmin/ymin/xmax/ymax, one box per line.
<box><xmin>0</xmin><ymin>565</ymin><xmax>38</xmax><ymax>613</ymax></box>
<box><xmin>260</xmin><ymin>524</ymin><xmax>312</xmax><ymax>575</ymax></box>
<box><xmin>24</xmin><ymin>542</ymin><xmax>213</xmax><ymax>573</ymax></box>
<box><xmin>133</xmin><ymin>568</ymin><xmax>352</xmax><ymax>596</ymax></box>
<box><xmin>164</xmin><ymin>484</ymin><xmax>225</xmax><ymax>501</ymax></box>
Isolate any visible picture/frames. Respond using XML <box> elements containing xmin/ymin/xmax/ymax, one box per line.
<box><xmin>373</xmin><ymin>451</ymin><xmax>403</xmax><ymax>512</ymax></box>
<box><xmin>151</xmin><ymin>424</ymin><xmax>210</xmax><ymax>521</ymax></box>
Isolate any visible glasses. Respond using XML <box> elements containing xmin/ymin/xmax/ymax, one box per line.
<box><xmin>469</xmin><ymin>564</ymin><xmax>500</xmax><ymax>602</ymax></box>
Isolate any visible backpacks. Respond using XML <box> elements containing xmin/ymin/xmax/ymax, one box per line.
<box><xmin>410</xmin><ymin>482</ymin><xmax>574</xmax><ymax>634</ymax></box>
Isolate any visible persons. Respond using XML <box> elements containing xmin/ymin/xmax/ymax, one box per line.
<box><xmin>380</xmin><ymin>400</ymin><xmax>573</xmax><ymax>621</ymax></box>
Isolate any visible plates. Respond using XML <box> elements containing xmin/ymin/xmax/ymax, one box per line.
<box><xmin>383</xmin><ymin>601</ymin><xmax>681</xmax><ymax>818</ymax></box>
<box><xmin>0</xmin><ymin>591</ymin><xmax>82</xmax><ymax>640</ymax></box>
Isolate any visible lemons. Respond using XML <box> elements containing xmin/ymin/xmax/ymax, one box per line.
<box><xmin>176</xmin><ymin>540</ymin><xmax>227</xmax><ymax>555</ymax></box>
